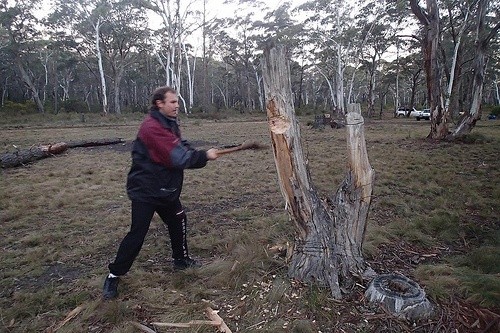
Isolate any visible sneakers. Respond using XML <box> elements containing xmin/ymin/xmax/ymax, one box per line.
<box><xmin>103</xmin><ymin>273</ymin><xmax>119</xmax><ymax>299</ymax></box>
<box><xmin>174</xmin><ymin>257</ymin><xmax>202</xmax><ymax>269</ymax></box>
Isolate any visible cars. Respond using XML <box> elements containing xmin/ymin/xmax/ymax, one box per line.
<box><xmin>417</xmin><ymin>109</ymin><xmax>431</xmax><ymax>120</ymax></box>
<box><xmin>488</xmin><ymin>114</ymin><xmax>496</xmax><ymax>120</ymax></box>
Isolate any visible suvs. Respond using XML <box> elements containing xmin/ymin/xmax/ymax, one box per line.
<box><xmin>396</xmin><ymin>106</ymin><xmax>422</xmax><ymax>118</ymax></box>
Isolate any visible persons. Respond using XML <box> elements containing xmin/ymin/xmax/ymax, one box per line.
<box><xmin>103</xmin><ymin>87</ymin><xmax>222</xmax><ymax>302</ymax></box>
